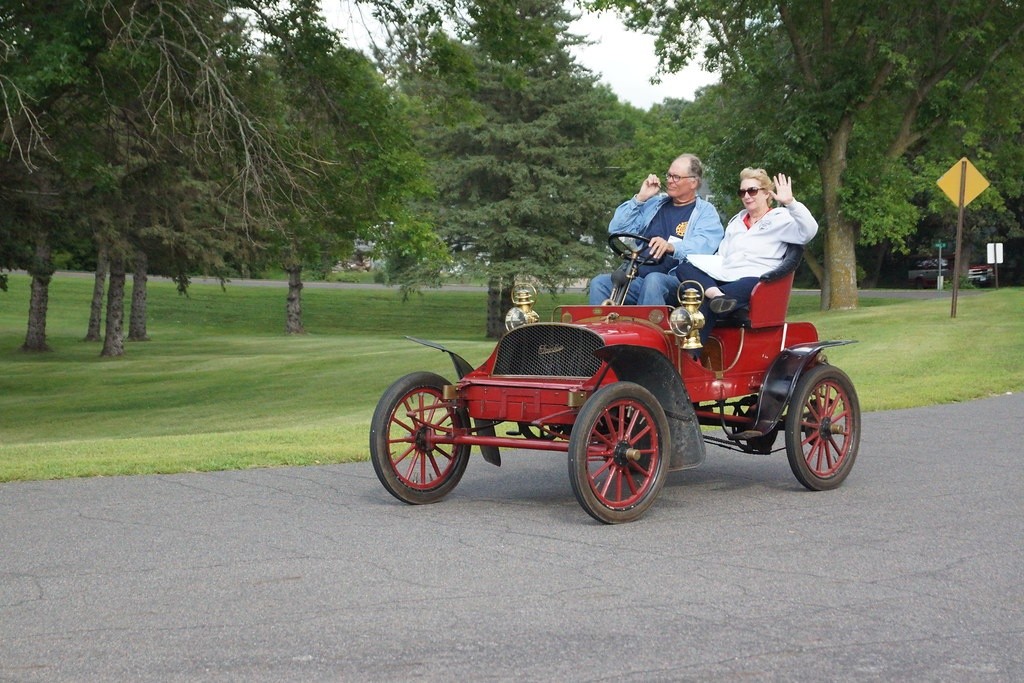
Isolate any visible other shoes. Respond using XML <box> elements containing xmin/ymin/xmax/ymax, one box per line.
<box><xmin>708</xmin><ymin>295</ymin><xmax>737</xmax><ymax>313</ymax></box>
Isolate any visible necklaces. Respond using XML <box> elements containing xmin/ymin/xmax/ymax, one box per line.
<box><xmin>657</xmin><ymin>181</ymin><xmax>702</xmax><ymax>205</ymax></box>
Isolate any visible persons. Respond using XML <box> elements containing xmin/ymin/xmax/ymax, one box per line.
<box><xmin>589</xmin><ymin>152</ymin><xmax>724</xmax><ymax>308</ymax></box>
<box><xmin>674</xmin><ymin>165</ymin><xmax>818</xmax><ymax>366</ymax></box>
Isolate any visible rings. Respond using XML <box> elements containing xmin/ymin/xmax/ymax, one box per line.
<box><xmin>783</xmin><ymin>183</ymin><xmax>788</xmax><ymax>185</ymax></box>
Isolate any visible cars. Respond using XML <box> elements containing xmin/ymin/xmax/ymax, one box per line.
<box><xmin>903</xmin><ymin>249</ymin><xmax>997</xmax><ymax>289</ymax></box>
<box><xmin>372</xmin><ymin>231</ymin><xmax>862</xmax><ymax>526</ymax></box>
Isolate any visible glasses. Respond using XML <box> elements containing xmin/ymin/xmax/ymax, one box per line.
<box><xmin>737</xmin><ymin>187</ymin><xmax>766</xmax><ymax>198</ymax></box>
<box><xmin>666</xmin><ymin>173</ymin><xmax>695</xmax><ymax>182</ymax></box>
